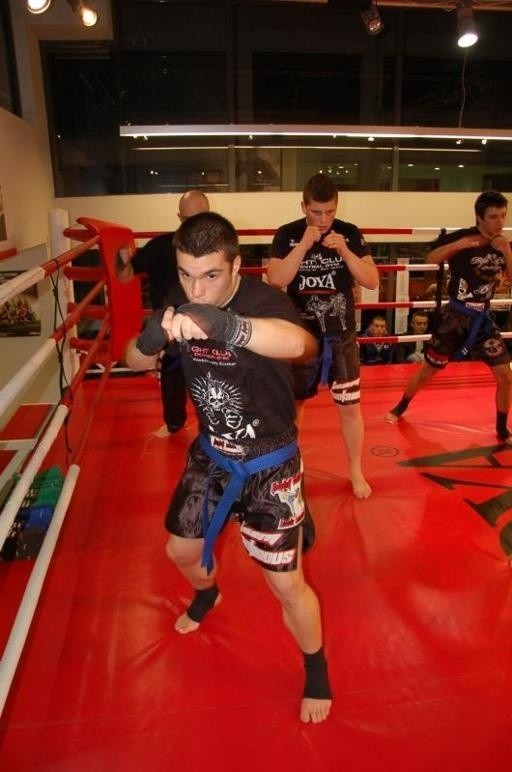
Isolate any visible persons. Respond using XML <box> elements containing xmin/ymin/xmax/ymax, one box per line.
<box><xmin>384</xmin><ymin>190</ymin><xmax>512</xmax><ymax>446</ymax></box>
<box><xmin>359</xmin><ymin>317</ymin><xmax>396</xmax><ymax>365</ymax></box>
<box><xmin>117</xmin><ymin>190</ymin><xmax>233</xmax><ymax>439</ymax></box>
<box><xmin>124</xmin><ymin>213</ymin><xmax>333</xmax><ymax>726</ymax></box>
<box><xmin>267</xmin><ymin>176</ymin><xmax>381</xmax><ymax>499</ymax></box>
<box><xmin>396</xmin><ymin>310</ymin><xmax>440</xmax><ymax>362</ymax></box>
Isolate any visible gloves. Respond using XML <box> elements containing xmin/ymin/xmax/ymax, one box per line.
<box><xmin>135</xmin><ymin>301</ymin><xmax>253</xmax><ymax>357</ymax></box>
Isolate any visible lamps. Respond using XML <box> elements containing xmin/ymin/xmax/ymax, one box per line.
<box><xmin>359</xmin><ymin>1</ymin><xmax>479</xmax><ymax>48</ymax></box>
<box><xmin>23</xmin><ymin>0</ymin><xmax>99</xmax><ymax>29</ymax></box>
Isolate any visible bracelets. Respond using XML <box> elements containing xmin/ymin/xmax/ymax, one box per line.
<box><xmin>224</xmin><ymin>310</ymin><xmax>251</xmax><ymax>351</ymax></box>
